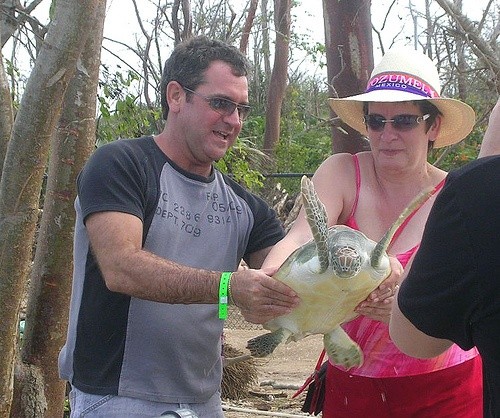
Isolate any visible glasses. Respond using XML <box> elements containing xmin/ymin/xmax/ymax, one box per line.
<box><xmin>363</xmin><ymin>111</ymin><xmax>433</xmax><ymax>131</ymax></box>
<box><xmin>185</xmin><ymin>88</ymin><xmax>252</xmax><ymax>122</ymax></box>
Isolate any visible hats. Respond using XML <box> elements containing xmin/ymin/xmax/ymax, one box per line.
<box><xmin>327</xmin><ymin>47</ymin><xmax>475</xmax><ymax>149</ymax></box>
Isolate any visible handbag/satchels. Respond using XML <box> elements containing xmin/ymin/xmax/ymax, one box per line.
<box><xmin>291</xmin><ymin>348</ymin><xmax>329</xmax><ymax>416</ymax></box>
